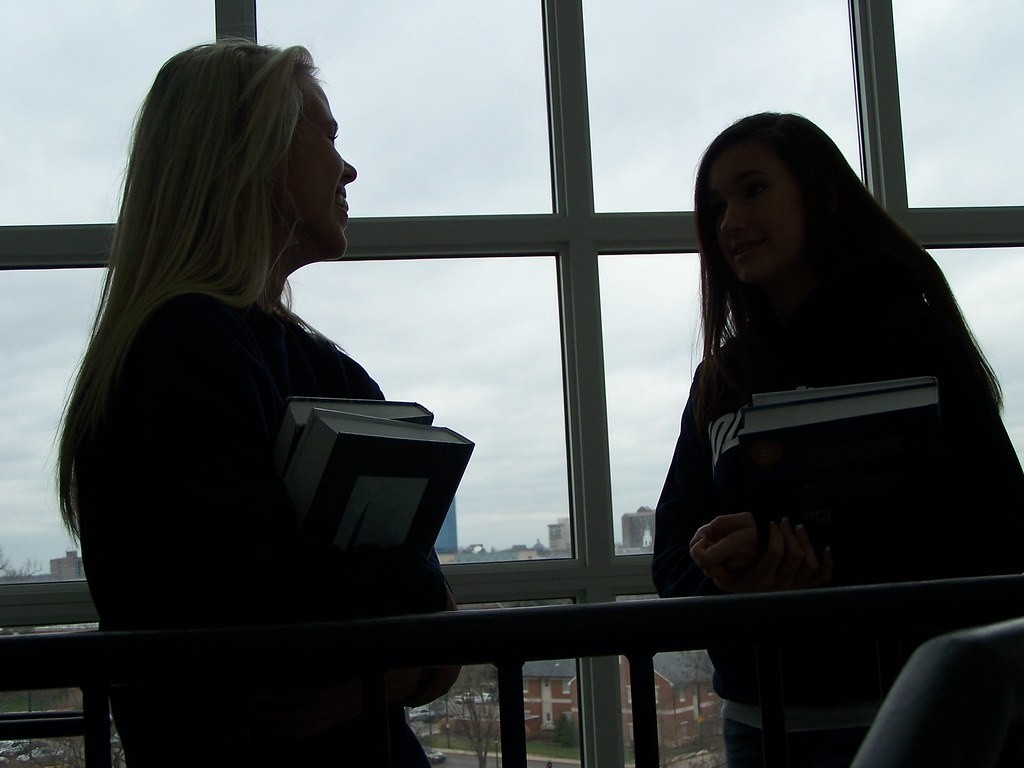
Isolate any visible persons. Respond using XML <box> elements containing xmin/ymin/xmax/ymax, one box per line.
<box><xmin>59</xmin><ymin>48</ymin><xmax>475</xmax><ymax>768</ymax></box>
<box><xmin>650</xmin><ymin>112</ymin><xmax>1023</xmax><ymax>768</ymax></box>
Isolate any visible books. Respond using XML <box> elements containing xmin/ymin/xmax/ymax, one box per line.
<box><xmin>737</xmin><ymin>375</ymin><xmax>943</xmax><ymax>644</ymax></box>
<box><xmin>271</xmin><ymin>395</ymin><xmax>475</xmax><ymax>561</ymax></box>
